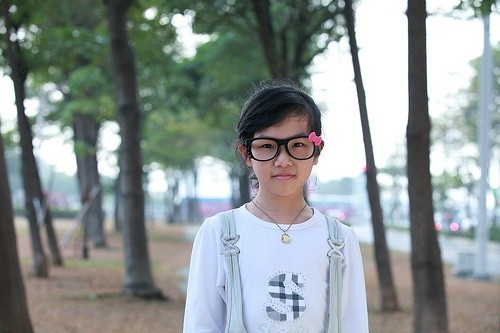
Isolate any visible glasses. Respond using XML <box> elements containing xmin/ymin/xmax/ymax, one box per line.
<box><xmin>245</xmin><ymin>131</ymin><xmax>322</xmax><ymax>161</ymax></box>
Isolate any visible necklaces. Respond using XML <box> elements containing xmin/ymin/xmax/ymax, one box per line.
<box><xmin>251</xmin><ymin>197</ymin><xmax>308</xmax><ymax>243</ymax></box>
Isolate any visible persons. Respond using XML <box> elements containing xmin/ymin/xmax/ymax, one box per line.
<box><xmin>183</xmin><ymin>85</ymin><xmax>369</xmax><ymax>333</ymax></box>
